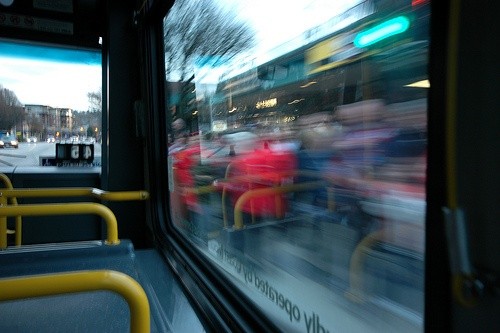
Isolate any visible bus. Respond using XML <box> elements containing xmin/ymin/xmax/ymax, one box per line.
<box><xmin>202</xmin><ymin>0</ymin><xmax>428</xmax><ymax>287</ymax></box>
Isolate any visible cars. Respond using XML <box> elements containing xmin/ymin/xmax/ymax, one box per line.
<box><xmin>0</xmin><ymin>128</ymin><xmax>97</xmax><ymax>148</ymax></box>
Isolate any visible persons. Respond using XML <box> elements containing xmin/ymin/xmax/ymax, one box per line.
<box><xmin>169</xmin><ymin>102</ymin><xmax>427</xmax><ymax>214</ymax></box>
